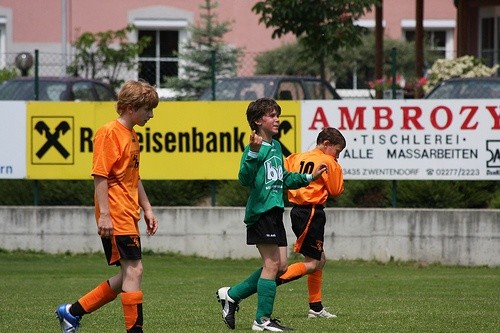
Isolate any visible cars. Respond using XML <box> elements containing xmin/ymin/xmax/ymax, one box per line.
<box><xmin>422</xmin><ymin>77</ymin><xmax>500</xmax><ymax>99</ymax></box>
<box><xmin>198</xmin><ymin>76</ymin><xmax>342</xmax><ymax>100</ymax></box>
<box><xmin>0</xmin><ymin>75</ymin><xmax>118</xmax><ymax>101</ymax></box>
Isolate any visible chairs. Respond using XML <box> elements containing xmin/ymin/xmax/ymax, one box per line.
<box><xmin>244</xmin><ymin>90</ymin><xmax>258</xmax><ymax>101</ymax></box>
<box><xmin>59</xmin><ymin>90</ymin><xmax>76</xmax><ymax>101</ymax></box>
<box><xmin>279</xmin><ymin>90</ymin><xmax>293</xmax><ymax>101</ymax></box>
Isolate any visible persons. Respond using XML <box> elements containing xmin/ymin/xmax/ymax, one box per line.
<box><xmin>275</xmin><ymin>128</ymin><xmax>346</xmax><ymax>319</ymax></box>
<box><xmin>216</xmin><ymin>97</ymin><xmax>326</xmax><ymax>332</ymax></box>
<box><xmin>55</xmin><ymin>80</ymin><xmax>159</xmax><ymax>333</ymax></box>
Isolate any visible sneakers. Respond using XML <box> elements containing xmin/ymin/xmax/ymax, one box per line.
<box><xmin>251</xmin><ymin>316</ymin><xmax>294</xmax><ymax>332</ymax></box>
<box><xmin>216</xmin><ymin>286</ymin><xmax>239</xmax><ymax>329</ymax></box>
<box><xmin>55</xmin><ymin>303</ymin><xmax>82</xmax><ymax>333</ymax></box>
<box><xmin>307</xmin><ymin>306</ymin><xmax>338</xmax><ymax>319</ymax></box>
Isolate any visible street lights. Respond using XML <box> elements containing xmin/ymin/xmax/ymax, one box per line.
<box><xmin>207</xmin><ymin>39</ymin><xmax>219</xmax><ymax>100</ymax></box>
<box><xmin>389</xmin><ymin>40</ymin><xmax>400</xmax><ymax>99</ymax></box>
